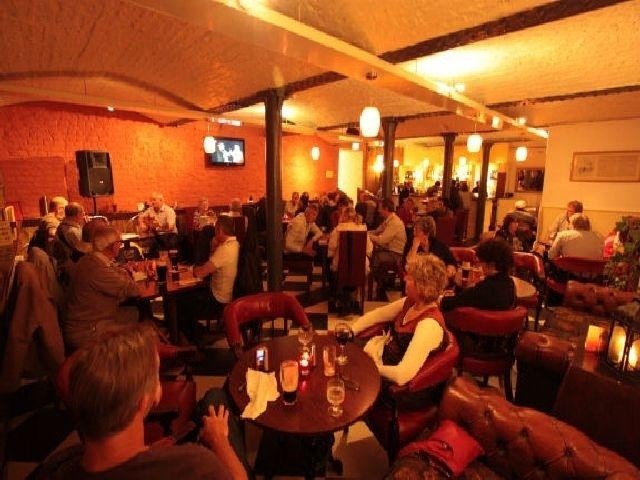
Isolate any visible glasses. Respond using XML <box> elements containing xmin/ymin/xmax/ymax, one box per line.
<box><xmin>341</xmin><ymin>373</ymin><xmax>360</xmax><ymax>391</ymax></box>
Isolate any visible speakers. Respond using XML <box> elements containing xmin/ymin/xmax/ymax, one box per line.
<box><xmin>75</xmin><ymin>150</ymin><xmax>115</xmax><ymax>198</ymax></box>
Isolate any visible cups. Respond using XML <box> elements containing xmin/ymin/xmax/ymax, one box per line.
<box><xmin>323</xmin><ymin>346</ymin><xmax>337</xmax><ymax>377</ymax></box>
<box><xmin>146</xmin><ymin>248</ymin><xmax>182</xmax><ymax>286</ymax></box>
<box><xmin>280</xmin><ymin>359</ymin><xmax>299</xmax><ymax>406</ymax></box>
<box><xmin>461</xmin><ymin>260</ymin><xmax>483</xmax><ymax>284</ymax></box>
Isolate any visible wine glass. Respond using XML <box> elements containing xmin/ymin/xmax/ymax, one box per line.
<box><xmin>321</xmin><ymin>226</ymin><xmax>328</xmax><ymax>240</ymax></box>
<box><xmin>334</xmin><ymin>324</ymin><xmax>351</xmax><ymax>365</ymax></box>
<box><xmin>297</xmin><ymin>323</ymin><xmax>313</xmax><ymax>350</ymax></box>
<box><xmin>326</xmin><ymin>377</ymin><xmax>345</xmax><ymax>419</ymax></box>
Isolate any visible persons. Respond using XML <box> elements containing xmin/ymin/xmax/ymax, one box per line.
<box><xmin>215</xmin><ymin>142</ymin><xmax>244</xmax><ymax>162</ymax></box>
<box><xmin>41</xmin><ymin>178</ymin><xmax>605</xmax><ymax>479</ymax></box>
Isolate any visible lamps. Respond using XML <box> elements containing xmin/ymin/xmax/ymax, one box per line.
<box><xmin>311</xmin><ymin>136</ymin><xmax>319</xmax><ymax>160</ymax></box>
<box><xmin>360</xmin><ymin>72</ymin><xmax>381</xmax><ymax>139</ymax></box>
<box><xmin>515</xmin><ymin>131</ymin><xmax>528</xmax><ymax>161</ymax></box>
<box><xmin>467</xmin><ymin>120</ymin><xmax>481</xmax><ymax>153</ymax></box>
<box><xmin>204</xmin><ymin>120</ymin><xmax>216</xmax><ymax>154</ymax></box>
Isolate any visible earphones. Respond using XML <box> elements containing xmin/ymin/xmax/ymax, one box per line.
<box><xmin>238</xmin><ymin>383</ymin><xmax>247</xmax><ymax>392</ymax></box>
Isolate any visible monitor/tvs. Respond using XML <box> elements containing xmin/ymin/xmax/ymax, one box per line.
<box><xmin>209</xmin><ymin>136</ymin><xmax>245</xmax><ymax>167</ymax></box>
